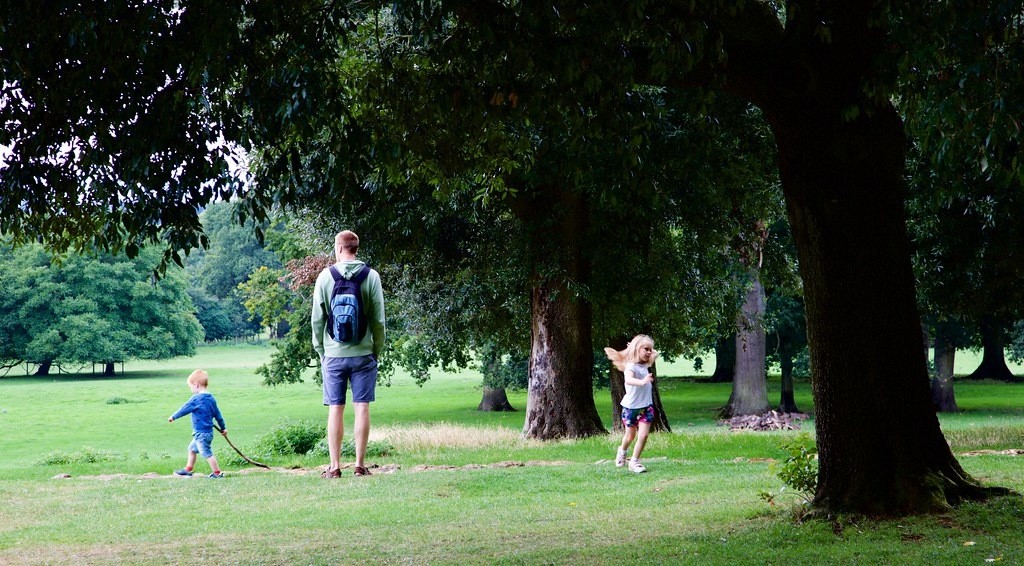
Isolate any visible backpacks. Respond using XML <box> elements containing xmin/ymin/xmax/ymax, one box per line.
<box><xmin>326</xmin><ymin>265</ymin><xmax>370</xmax><ymax>346</ymax></box>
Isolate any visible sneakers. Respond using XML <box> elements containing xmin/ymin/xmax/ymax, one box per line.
<box><xmin>629</xmin><ymin>461</ymin><xmax>647</xmax><ymax>473</ymax></box>
<box><xmin>616</xmin><ymin>446</ymin><xmax>628</xmax><ymax>467</ymax></box>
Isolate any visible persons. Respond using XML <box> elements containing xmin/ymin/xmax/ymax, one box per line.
<box><xmin>168</xmin><ymin>370</ymin><xmax>228</xmax><ymax>478</ymax></box>
<box><xmin>604</xmin><ymin>334</ymin><xmax>658</xmax><ymax>474</ymax></box>
<box><xmin>311</xmin><ymin>229</ymin><xmax>386</xmax><ymax>476</ymax></box>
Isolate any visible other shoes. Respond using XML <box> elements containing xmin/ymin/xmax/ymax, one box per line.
<box><xmin>205</xmin><ymin>472</ymin><xmax>223</xmax><ymax>478</ymax></box>
<box><xmin>173</xmin><ymin>469</ymin><xmax>192</xmax><ymax>477</ymax></box>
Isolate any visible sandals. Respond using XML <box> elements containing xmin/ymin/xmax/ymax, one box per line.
<box><xmin>321</xmin><ymin>467</ymin><xmax>341</xmax><ymax>478</ymax></box>
<box><xmin>354</xmin><ymin>467</ymin><xmax>372</xmax><ymax>476</ymax></box>
